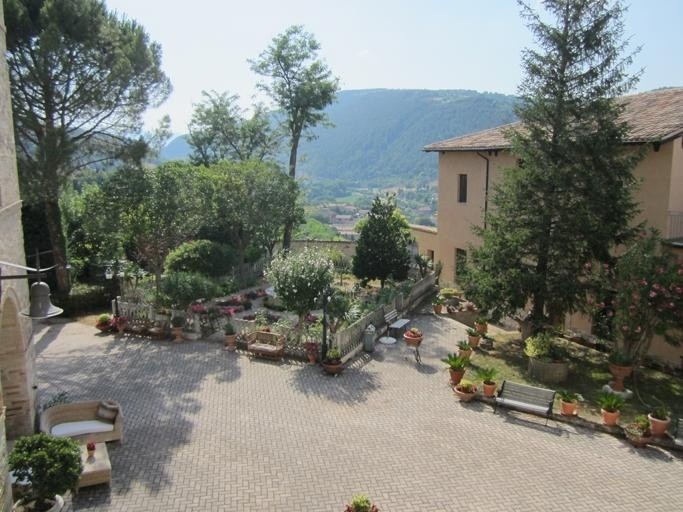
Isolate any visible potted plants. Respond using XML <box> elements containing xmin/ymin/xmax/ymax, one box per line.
<box><xmin>222</xmin><ymin>322</ymin><xmax>239</xmax><ymax>352</ymax></box>
<box><xmin>3</xmin><ymin>432</ymin><xmax>84</xmax><ymax>512</ymax></box>
<box><xmin>440</xmin><ymin>316</ymin><xmax>497</xmax><ymax>402</ymax></box>
<box><xmin>321</xmin><ymin>347</ymin><xmax>344</xmax><ymax>375</ymax></box>
<box><xmin>559</xmin><ymin>387</ymin><xmax>672</xmax><ymax>448</ymax></box>
<box><xmin>431</xmin><ymin>288</ymin><xmax>473</xmax><ymax>314</ymax></box>
<box><xmin>170</xmin><ymin>316</ymin><xmax>186</xmax><ymax>343</ymax></box>
<box><xmin>522</xmin><ymin>329</ymin><xmax>575</xmax><ymax>383</ymax></box>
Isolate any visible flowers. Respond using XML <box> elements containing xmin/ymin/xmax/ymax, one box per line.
<box><xmin>404</xmin><ymin>327</ymin><xmax>422</xmax><ymax>337</ymax></box>
<box><xmin>95</xmin><ymin>303</ymin><xmax>128</xmax><ymax>327</ymax></box>
<box><xmin>577</xmin><ymin>226</ymin><xmax>683</xmax><ymax>365</ymax></box>
<box><xmin>86</xmin><ymin>439</ymin><xmax>96</xmax><ymax>450</ymax></box>
<box><xmin>302</xmin><ymin>340</ymin><xmax>321</xmax><ymax>354</ymax></box>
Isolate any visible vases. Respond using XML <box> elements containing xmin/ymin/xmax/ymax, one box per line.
<box><xmin>307</xmin><ymin>354</ymin><xmax>318</xmax><ymax>365</ymax></box>
<box><xmin>606</xmin><ymin>365</ymin><xmax>636</xmax><ymax>391</ymax></box>
<box><xmin>87</xmin><ymin>450</ymin><xmax>93</xmax><ymax>457</ymax></box>
<box><xmin>94</xmin><ymin>327</ymin><xmax>128</xmax><ymax>335</ymax></box>
<box><xmin>405</xmin><ymin>338</ymin><xmax>422</xmax><ymax>347</ymax></box>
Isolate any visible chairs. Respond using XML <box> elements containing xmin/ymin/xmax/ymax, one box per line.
<box><xmin>130</xmin><ymin>318</ymin><xmax>168</xmax><ymax>341</ymax></box>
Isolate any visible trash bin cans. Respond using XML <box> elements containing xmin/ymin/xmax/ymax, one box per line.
<box><xmin>363</xmin><ymin>324</ymin><xmax>376</xmax><ymax>352</ymax></box>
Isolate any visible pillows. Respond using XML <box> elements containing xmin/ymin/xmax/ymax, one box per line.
<box><xmin>95</xmin><ymin>399</ymin><xmax>119</xmax><ymax>425</ymax></box>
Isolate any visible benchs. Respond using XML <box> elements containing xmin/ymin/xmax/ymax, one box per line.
<box><xmin>245</xmin><ymin>331</ymin><xmax>286</xmax><ymax>361</ymax></box>
<box><xmin>493</xmin><ymin>380</ymin><xmax>557</xmax><ymax>426</ymax></box>
<box><xmin>383</xmin><ymin>309</ymin><xmax>410</xmax><ymax>340</ymax></box>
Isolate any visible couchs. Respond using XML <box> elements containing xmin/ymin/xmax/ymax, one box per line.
<box><xmin>37</xmin><ymin>397</ymin><xmax>123</xmax><ymax>447</ymax></box>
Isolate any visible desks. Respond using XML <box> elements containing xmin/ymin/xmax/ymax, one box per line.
<box><xmin>65</xmin><ymin>442</ymin><xmax>112</xmax><ymax>494</ymax></box>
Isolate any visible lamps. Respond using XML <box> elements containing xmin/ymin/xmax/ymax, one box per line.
<box><xmin>0</xmin><ymin>250</ymin><xmax>64</xmax><ymax>319</ymax></box>
